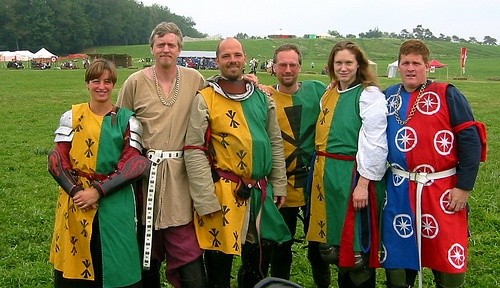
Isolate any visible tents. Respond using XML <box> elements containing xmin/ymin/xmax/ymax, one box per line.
<box><xmin>0</xmin><ymin>48</ymin><xmax>58</xmax><ymax>69</ymax></box>
<box><xmin>178</xmin><ymin>51</ymin><xmax>216</xmax><ymax>58</ymax></box>
<box><xmin>367</xmin><ymin>59</ymin><xmax>449</xmax><ymax>82</ymax></box>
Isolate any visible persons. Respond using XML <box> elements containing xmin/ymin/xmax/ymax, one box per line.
<box><xmin>7</xmin><ymin>57</ymin><xmax>96</xmax><ymax>69</ymax></box>
<box><xmin>179</xmin><ymin>39</ymin><xmax>288</xmax><ymax>288</ymax></box>
<box><xmin>48</xmin><ymin>59</ymin><xmax>148</xmax><ymax>288</ymax></box>
<box><xmin>115</xmin><ymin>22</ymin><xmax>260</xmax><ymax>288</ymax></box>
<box><xmin>381</xmin><ymin>42</ymin><xmax>489</xmax><ymax>288</ymax></box>
<box><xmin>257</xmin><ymin>43</ymin><xmax>336</xmax><ymax>288</ymax></box>
<box><xmin>176</xmin><ymin>57</ymin><xmax>276</xmax><ymax>76</ymax></box>
<box><xmin>304</xmin><ymin>41</ymin><xmax>389</xmax><ymax>288</ymax></box>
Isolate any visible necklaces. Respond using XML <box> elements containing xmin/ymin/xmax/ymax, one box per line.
<box><xmin>152</xmin><ymin>64</ymin><xmax>181</xmax><ymax>107</ymax></box>
<box><xmin>395</xmin><ymin>80</ymin><xmax>427</xmax><ymax>125</ymax></box>
<box><xmin>336</xmin><ymin>83</ymin><xmax>360</xmax><ymax>94</ymax></box>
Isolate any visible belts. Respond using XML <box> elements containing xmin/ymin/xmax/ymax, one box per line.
<box><xmin>390</xmin><ymin>164</ymin><xmax>457</xmax><ymax>288</ymax></box>
<box><xmin>286</xmin><ymin>165</ymin><xmax>311</xmax><ymax>180</ymax></box>
<box><xmin>214</xmin><ymin>167</ymin><xmax>269</xmax><ymax>280</ymax></box>
<box><xmin>140</xmin><ymin>148</ymin><xmax>184</xmax><ymax>272</ymax></box>
<box><xmin>70</xmin><ymin>168</ymin><xmax>107</xmax><ymax>184</ymax></box>
